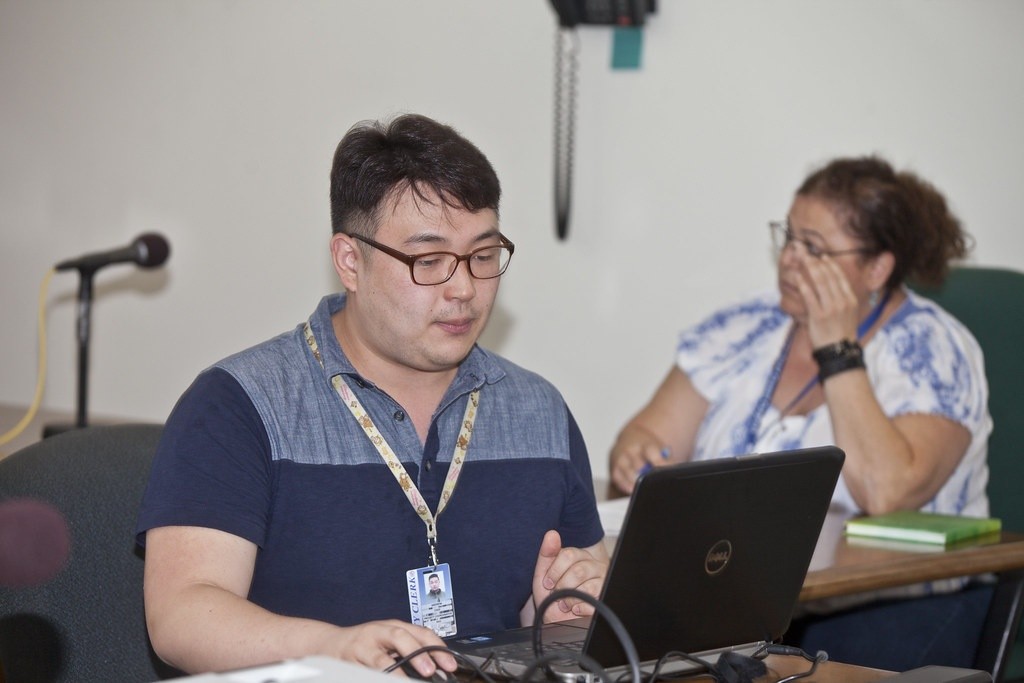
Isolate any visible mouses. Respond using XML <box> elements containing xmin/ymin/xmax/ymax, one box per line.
<box><xmin>394</xmin><ymin>654</ymin><xmax>458</xmax><ymax>683</ymax></box>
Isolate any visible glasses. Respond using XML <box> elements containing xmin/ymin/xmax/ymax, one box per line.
<box><xmin>769</xmin><ymin>220</ymin><xmax>871</xmax><ymax>261</ymax></box>
<box><xmin>349</xmin><ymin>233</ymin><xmax>514</xmax><ymax>286</ymax></box>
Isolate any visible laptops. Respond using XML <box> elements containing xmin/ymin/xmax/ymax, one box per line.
<box><xmin>435</xmin><ymin>447</ymin><xmax>847</xmax><ymax>683</ymax></box>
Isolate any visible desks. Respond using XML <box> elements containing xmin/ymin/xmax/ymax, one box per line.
<box><xmin>606</xmin><ymin>484</ymin><xmax>1024</xmax><ymax>683</ymax></box>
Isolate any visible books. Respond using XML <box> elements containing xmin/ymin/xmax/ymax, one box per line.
<box><xmin>840</xmin><ymin>511</ymin><xmax>1002</xmax><ymax>545</ymax></box>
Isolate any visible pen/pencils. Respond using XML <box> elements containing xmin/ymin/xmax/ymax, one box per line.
<box><xmin>634</xmin><ymin>448</ymin><xmax>668</xmax><ymax>484</ymax></box>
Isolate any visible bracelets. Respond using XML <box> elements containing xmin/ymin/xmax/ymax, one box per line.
<box><xmin>812</xmin><ymin>339</ymin><xmax>866</xmax><ymax>385</ymax></box>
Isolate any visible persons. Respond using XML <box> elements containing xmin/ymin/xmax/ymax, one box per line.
<box><xmin>136</xmin><ymin>113</ymin><xmax>610</xmax><ymax>678</ymax></box>
<box><xmin>425</xmin><ymin>574</ymin><xmax>445</xmax><ymax>599</ymax></box>
<box><xmin>610</xmin><ymin>154</ymin><xmax>997</xmax><ymax>673</ymax></box>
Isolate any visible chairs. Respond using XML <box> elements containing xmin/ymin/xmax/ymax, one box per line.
<box><xmin>906</xmin><ymin>268</ymin><xmax>1024</xmax><ymax>682</ymax></box>
<box><xmin>0</xmin><ymin>416</ymin><xmax>185</xmax><ymax>683</ymax></box>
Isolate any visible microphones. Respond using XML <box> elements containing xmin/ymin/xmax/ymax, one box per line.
<box><xmin>55</xmin><ymin>232</ymin><xmax>169</xmax><ymax>272</ymax></box>
